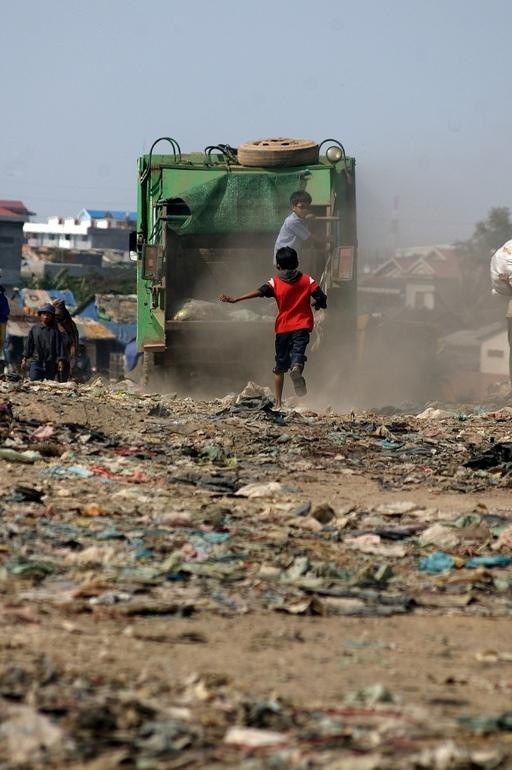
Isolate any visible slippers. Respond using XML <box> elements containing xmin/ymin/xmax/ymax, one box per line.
<box><xmin>289</xmin><ymin>368</ymin><xmax>308</xmax><ymax>398</ymax></box>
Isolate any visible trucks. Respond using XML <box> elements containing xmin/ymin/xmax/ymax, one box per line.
<box><xmin>141</xmin><ymin>139</ymin><xmax>356</xmax><ymax>395</ymax></box>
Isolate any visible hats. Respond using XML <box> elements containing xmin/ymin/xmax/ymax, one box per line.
<box><xmin>36</xmin><ymin>298</ymin><xmax>66</xmax><ymax>317</ymax></box>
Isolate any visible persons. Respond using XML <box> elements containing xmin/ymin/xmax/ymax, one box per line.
<box><xmin>215</xmin><ymin>245</ymin><xmax>329</xmax><ymax>412</ymax></box>
<box><xmin>487</xmin><ymin>237</ymin><xmax>511</xmax><ymax>385</ymax></box>
<box><xmin>0</xmin><ymin>284</ymin><xmax>94</xmax><ymax>383</ymax></box>
<box><xmin>270</xmin><ymin>189</ymin><xmax>338</xmax><ymax>276</ymax></box>
